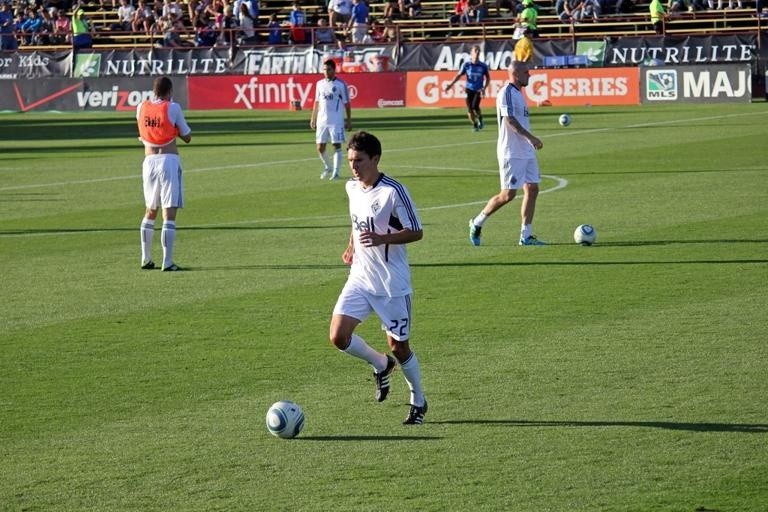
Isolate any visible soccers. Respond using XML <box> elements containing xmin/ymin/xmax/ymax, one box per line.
<box><xmin>574</xmin><ymin>224</ymin><xmax>597</xmax><ymax>245</ymax></box>
<box><xmin>266</xmin><ymin>400</ymin><xmax>304</xmax><ymax>438</ymax></box>
<box><xmin>559</xmin><ymin>115</ymin><xmax>570</xmax><ymax>126</ymax></box>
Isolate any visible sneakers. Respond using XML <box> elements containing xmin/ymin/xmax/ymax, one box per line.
<box><xmin>469</xmin><ymin>215</ymin><xmax>482</xmax><ymax>246</ymax></box>
<box><xmin>320</xmin><ymin>165</ymin><xmax>333</xmax><ymax>180</ymax></box>
<box><xmin>373</xmin><ymin>352</ymin><xmax>397</xmax><ymax>402</ymax></box>
<box><xmin>162</xmin><ymin>263</ymin><xmax>182</xmax><ymax>271</ymax></box>
<box><xmin>402</xmin><ymin>399</ymin><xmax>427</xmax><ymax>424</ymax></box>
<box><xmin>141</xmin><ymin>260</ymin><xmax>160</xmax><ymax>269</ymax></box>
<box><xmin>329</xmin><ymin>173</ymin><xmax>340</xmax><ymax>180</ymax></box>
<box><xmin>518</xmin><ymin>235</ymin><xmax>547</xmax><ymax>245</ymax></box>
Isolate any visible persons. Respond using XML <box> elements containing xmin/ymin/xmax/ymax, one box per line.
<box><xmin>329</xmin><ymin>131</ymin><xmax>428</xmax><ymax>425</ymax></box>
<box><xmin>444</xmin><ymin>45</ymin><xmax>490</xmax><ymax>131</ymax></box>
<box><xmin>514</xmin><ymin>30</ymin><xmax>535</xmax><ymax>70</ymax></box>
<box><xmin>555</xmin><ymin>0</ymin><xmax>767</xmax><ymax>35</ymax></box>
<box><xmin>310</xmin><ymin>58</ymin><xmax>352</xmax><ymax>181</ymax></box>
<box><xmin>136</xmin><ymin>77</ymin><xmax>191</xmax><ymax>272</ymax></box>
<box><xmin>469</xmin><ymin>61</ymin><xmax>546</xmax><ymax>247</ymax></box>
<box><xmin>450</xmin><ymin>0</ymin><xmax>538</xmax><ymax>38</ymax></box>
<box><xmin>1</xmin><ymin>1</ymin><xmax>421</xmax><ymax>54</ymax></box>
